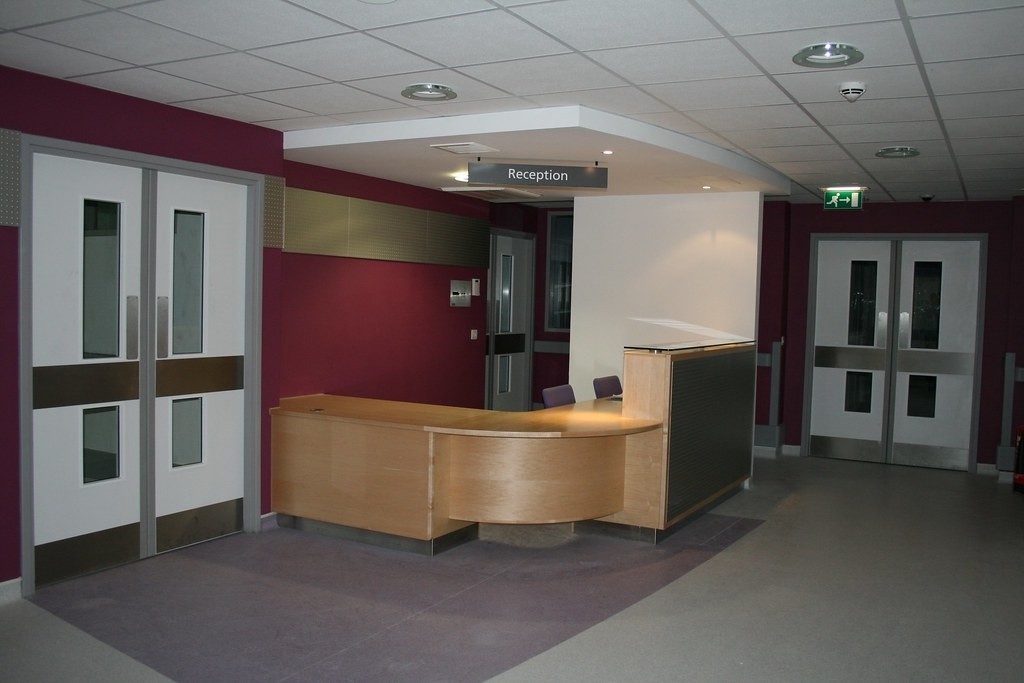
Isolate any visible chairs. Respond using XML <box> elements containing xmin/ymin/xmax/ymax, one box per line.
<box><xmin>541</xmin><ymin>384</ymin><xmax>576</xmax><ymax>408</ymax></box>
<box><xmin>594</xmin><ymin>375</ymin><xmax>623</xmax><ymax>399</ymax></box>
